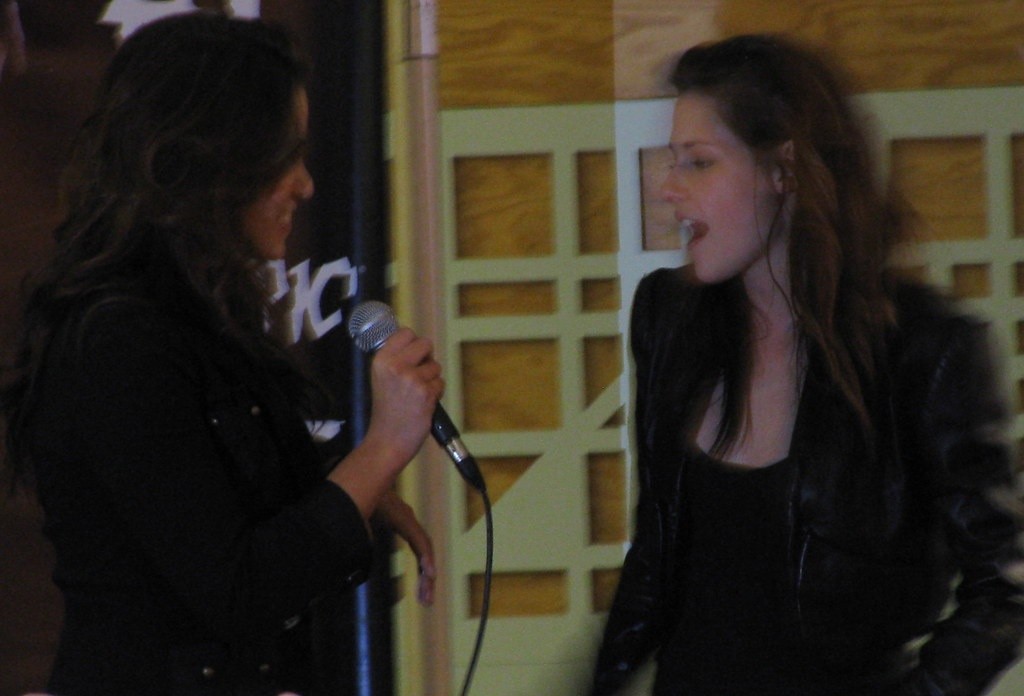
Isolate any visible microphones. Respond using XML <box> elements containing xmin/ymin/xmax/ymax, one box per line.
<box><xmin>349</xmin><ymin>299</ymin><xmax>487</xmax><ymax>497</ymax></box>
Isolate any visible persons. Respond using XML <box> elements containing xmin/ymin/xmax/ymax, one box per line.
<box><xmin>586</xmin><ymin>33</ymin><xmax>1024</xmax><ymax>696</ymax></box>
<box><xmin>17</xmin><ymin>11</ymin><xmax>444</xmax><ymax>696</ymax></box>
